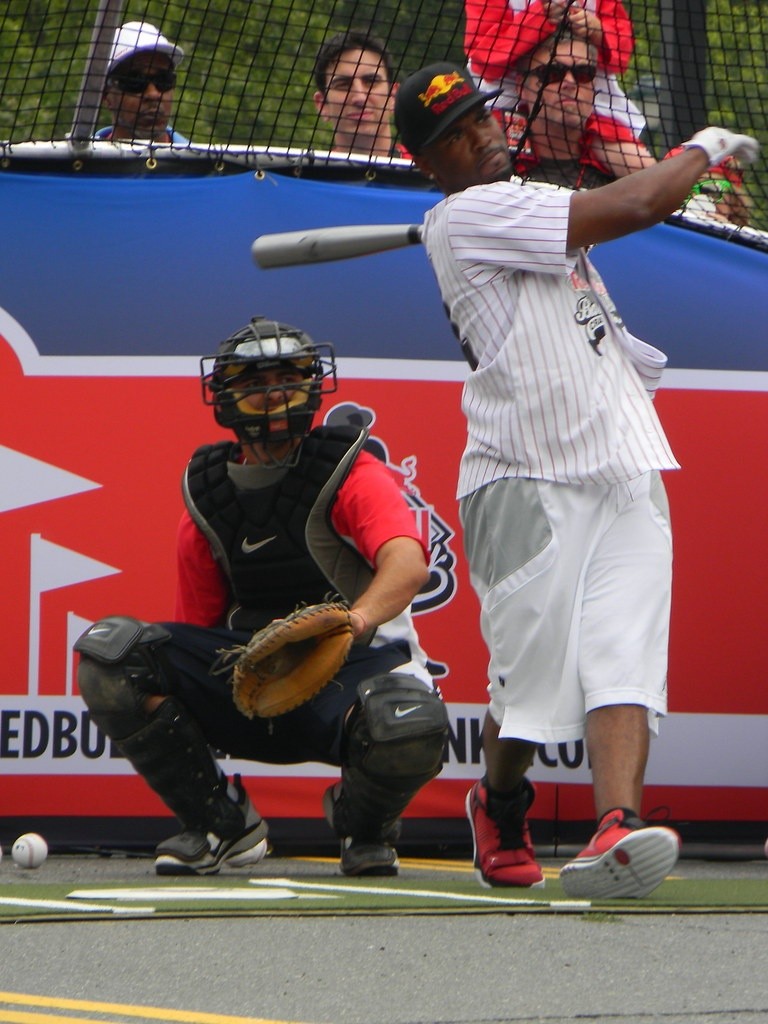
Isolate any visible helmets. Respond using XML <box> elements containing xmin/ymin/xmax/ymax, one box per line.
<box><xmin>200</xmin><ymin>316</ymin><xmax>338</xmax><ymax>470</ymax></box>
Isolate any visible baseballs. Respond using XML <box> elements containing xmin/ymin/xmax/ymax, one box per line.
<box><xmin>11</xmin><ymin>832</ymin><xmax>49</xmax><ymax>868</ymax></box>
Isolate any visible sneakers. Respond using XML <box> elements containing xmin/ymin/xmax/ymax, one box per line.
<box><xmin>465</xmin><ymin>774</ymin><xmax>546</xmax><ymax>890</ymax></box>
<box><xmin>154</xmin><ymin>773</ymin><xmax>267</xmax><ymax>876</ymax></box>
<box><xmin>322</xmin><ymin>784</ymin><xmax>399</xmax><ymax>875</ymax></box>
<box><xmin>560</xmin><ymin>805</ymin><xmax>687</xmax><ymax>901</ymax></box>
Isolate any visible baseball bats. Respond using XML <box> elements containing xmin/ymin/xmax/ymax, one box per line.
<box><xmin>251</xmin><ymin>224</ymin><xmax>421</xmax><ymax>271</ymax></box>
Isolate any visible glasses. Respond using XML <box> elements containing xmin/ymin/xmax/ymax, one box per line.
<box><xmin>521</xmin><ymin>62</ymin><xmax>598</xmax><ymax>84</ymax></box>
<box><xmin>678</xmin><ymin>179</ymin><xmax>735</xmax><ymax>209</ymax></box>
<box><xmin>109</xmin><ymin>70</ymin><xmax>176</xmax><ymax>92</ymax></box>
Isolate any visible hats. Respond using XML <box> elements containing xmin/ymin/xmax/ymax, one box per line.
<box><xmin>106</xmin><ymin>21</ymin><xmax>183</xmax><ymax>74</ymax></box>
<box><xmin>394</xmin><ymin>62</ymin><xmax>504</xmax><ymax>148</ymax></box>
<box><xmin>662</xmin><ymin>145</ymin><xmax>742</xmax><ymax>184</ymax></box>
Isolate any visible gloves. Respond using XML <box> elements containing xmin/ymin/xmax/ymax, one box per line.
<box><xmin>683</xmin><ymin>126</ymin><xmax>758</xmax><ymax>167</ymax></box>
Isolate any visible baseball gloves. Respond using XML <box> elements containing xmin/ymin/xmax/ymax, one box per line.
<box><xmin>230</xmin><ymin>602</ymin><xmax>358</xmax><ymax>720</ymax></box>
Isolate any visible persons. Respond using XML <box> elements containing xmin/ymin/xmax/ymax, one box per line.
<box><xmin>397</xmin><ymin>63</ymin><xmax>757</xmax><ymax>899</ymax></box>
<box><xmin>665</xmin><ymin>147</ymin><xmax>749</xmax><ymax>227</ymax></box>
<box><xmin>93</xmin><ymin>20</ymin><xmax>193</xmax><ymax>144</ymax></box>
<box><xmin>313</xmin><ymin>32</ymin><xmax>413</xmax><ymax>159</ymax></box>
<box><xmin>75</xmin><ymin>321</ymin><xmax>449</xmax><ymax>874</ymax></box>
<box><xmin>465</xmin><ymin>0</ymin><xmax>658</xmax><ymax>194</ymax></box>
